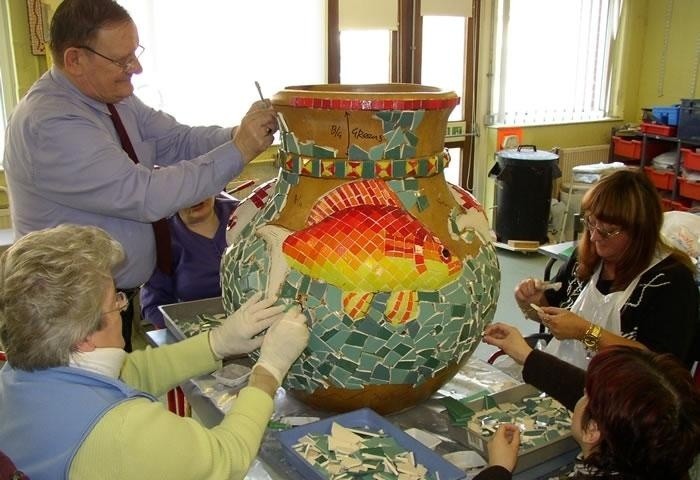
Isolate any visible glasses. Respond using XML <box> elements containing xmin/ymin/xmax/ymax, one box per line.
<box><xmin>579</xmin><ymin>216</ymin><xmax>621</xmax><ymax>242</ymax></box>
<box><xmin>77</xmin><ymin>42</ymin><xmax>145</xmax><ymax>73</ymax></box>
<box><xmin>101</xmin><ymin>292</ymin><xmax>130</xmax><ymax>316</ymax></box>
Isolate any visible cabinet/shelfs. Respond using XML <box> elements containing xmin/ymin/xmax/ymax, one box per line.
<box><xmin>608</xmin><ymin>126</ymin><xmax>700</xmax><ymax>211</ymax></box>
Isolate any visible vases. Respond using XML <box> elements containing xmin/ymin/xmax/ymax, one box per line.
<box><xmin>218</xmin><ymin>82</ymin><xmax>503</xmax><ymax>417</ymax></box>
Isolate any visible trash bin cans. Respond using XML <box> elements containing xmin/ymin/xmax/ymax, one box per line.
<box><xmin>487</xmin><ymin>144</ymin><xmax>562</xmax><ymax>246</ymax></box>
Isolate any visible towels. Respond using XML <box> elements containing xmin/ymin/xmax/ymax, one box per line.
<box><xmin>572</xmin><ymin>161</ymin><xmax>625</xmax><ymax>183</ymax></box>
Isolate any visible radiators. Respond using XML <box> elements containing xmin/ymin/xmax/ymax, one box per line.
<box><xmin>559</xmin><ymin>143</ymin><xmax>610</xmax><ymax>185</ymax></box>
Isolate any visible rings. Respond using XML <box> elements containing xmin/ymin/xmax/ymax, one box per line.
<box><xmin>264</xmin><ymin>127</ymin><xmax>273</xmax><ymax>135</ymax></box>
<box><xmin>542</xmin><ymin>315</ymin><xmax>551</xmax><ymax>324</ymax></box>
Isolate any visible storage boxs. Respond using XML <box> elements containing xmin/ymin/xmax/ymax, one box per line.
<box><xmin>612</xmin><ymin>103</ymin><xmax>700</xmax><ymax>214</ymax></box>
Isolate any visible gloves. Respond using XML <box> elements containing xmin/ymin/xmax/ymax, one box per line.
<box><xmin>250</xmin><ymin>303</ymin><xmax>310</xmax><ymax>384</ymax></box>
<box><xmin>207</xmin><ymin>290</ymin><xmax>285</xmax><ymax>360</ymax></box>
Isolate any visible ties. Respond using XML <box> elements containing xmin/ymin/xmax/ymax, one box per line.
<box><xmin>108</xmin><ymin>104</ymin><xmax>171</xmax><ymax>274</ymax></box>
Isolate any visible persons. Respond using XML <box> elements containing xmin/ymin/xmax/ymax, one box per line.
<box><xmin>138</xmin><ymin>195</ymin><xmax>242</xmax><ymax>329</ymax></box>
<box><xmin>471</xmin><ymin>321</ymin><xmax>700</xmax><ymax>479</ymax></box>
<box><xmin>1</xmin><ymin>222</ymin><xmax>310</xmax><ymax>479</ymax></box>
<box><xmin>3</xmin><ymin>1</ymin><xmax>281</xmax><ymax>353</ymax></box>
<box><xmin>514</xmin><ymin>168</ymin><xmax>699</xmax><ymax>378</ymax></box>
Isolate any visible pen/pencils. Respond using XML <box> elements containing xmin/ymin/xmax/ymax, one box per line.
<box><xmin>255</xmin><ymin>81</ymin><xmax>265</xmax><ymax>102</ymax></box>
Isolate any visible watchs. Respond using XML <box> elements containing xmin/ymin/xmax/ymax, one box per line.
<box><xmin>583</xmin><ymin>323</ymin><xmax>602</xmax><ymax>352</ymax></box>
